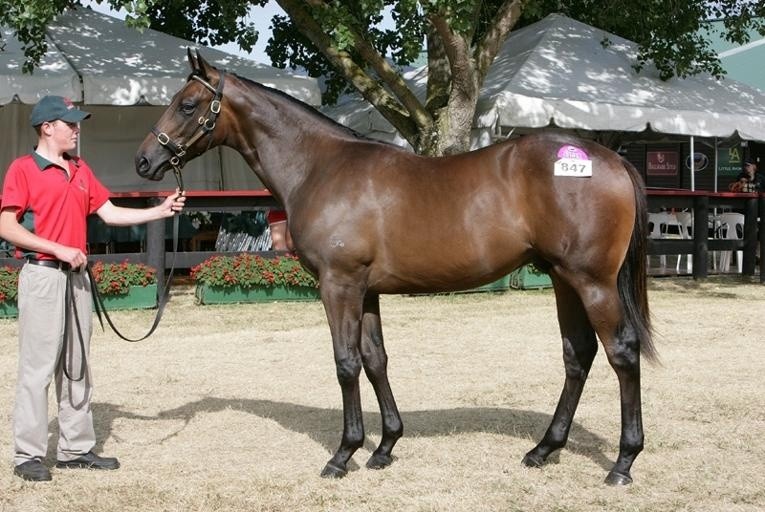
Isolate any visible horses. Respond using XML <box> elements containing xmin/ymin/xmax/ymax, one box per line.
<box><xmin>132</xmin><ymin>46</ymin><xmax>659</xmax><ymax>488</ymax></box>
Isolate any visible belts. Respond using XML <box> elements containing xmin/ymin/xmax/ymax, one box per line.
<box><xmin>24</xmin><ymin>255</ymin><xmax>94</xmax><ymax>272</ymax></box>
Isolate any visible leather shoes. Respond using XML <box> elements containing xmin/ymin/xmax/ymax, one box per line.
<box><xmin>14</xmin><ymin>456</ymin><xmax>53</xmax><ymax>482</ymax></box>
<box><xmin>56</xmin><ymin>450</ymin><xmax>120</xmax><ymax>471</ymax></box>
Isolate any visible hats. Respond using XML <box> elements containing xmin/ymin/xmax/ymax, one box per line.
<box><xmin>31</xmin><ymin>95</ymin><xmax>92</xmax><ymax>127</ymax></box>
<box><xmin>743</xmin><ymin>158</ymin><xmax>756</xmax><ymax>165</ymax></box>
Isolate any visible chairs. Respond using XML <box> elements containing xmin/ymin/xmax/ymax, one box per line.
<box><xmin>642</xmin><ymin>207</ymin><xmax>748</xmax><ymax>279</ymax></box>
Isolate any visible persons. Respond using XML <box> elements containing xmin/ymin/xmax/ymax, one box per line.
<box><xmin>266</xmin><ymin>192</ymin><xmax>295</xmax><ymax>252</ymax></box>
<box><xmin>0</xmin><ymin>94</ymin><xmax>187</xmax><ymax>483</ymax></box>
<box><xmin>738</xmin><ymin>156</ymin><xmax>765</xmax><ymax>194</ymax></box>
<box><xmin>656</xmin><ymin>152</ymin><xmax>674</xmax><ymax>169</ymax></box>
